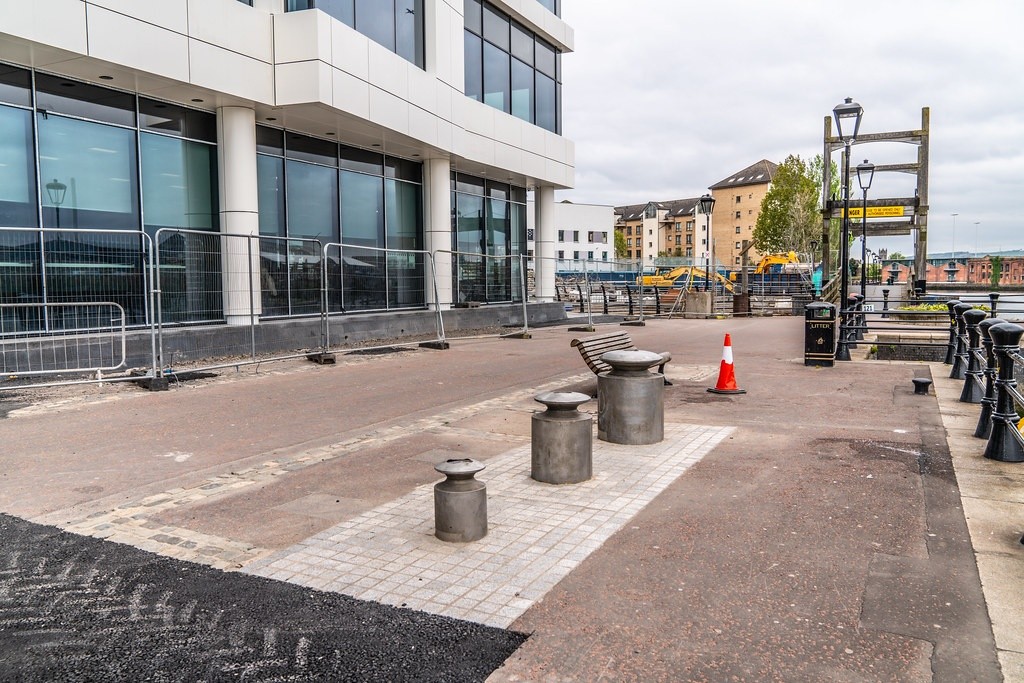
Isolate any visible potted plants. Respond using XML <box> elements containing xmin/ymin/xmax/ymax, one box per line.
<box><xmin>888</xmin><ymin>303</ymin><xmax>991</xmax><ymax>326</ymax></box>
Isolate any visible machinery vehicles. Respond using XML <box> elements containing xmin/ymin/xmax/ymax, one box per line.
<box><xmin>726</xmin><ymin>250</ymin><xmax>800</xmax><ymax>283</ymax></box>
<box><xmin>636</xmin><ymin>266</ymin><xmax>753</xmax><ymax>299</ymax></box>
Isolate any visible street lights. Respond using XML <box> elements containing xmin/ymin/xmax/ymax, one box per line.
<box><xmin>810</xmin><ymin>239</ymin><xmax>818</xmax><ymax>274</ymax></box>
<box><xmin>856</xmin><ymin>159</ymin><xmax>876</xmax><ymax>334</ymax></box>
<box><xmin>700</xmin><ymin>192</ymin><xmax>716</xmax><ymax>292</ymax></box>
<box><xmin>973</xmin><ymin>220</ymin><xmax>980</xmax><ymax>258</ymax></box>
<box><xmin>865</xmin><ymin>248</ymin><xmax>882</xmax><ymax>284</ymax></box>
<box><xmin>950</xmin><ymin>213</ymin><xmax>959</xmax><ymax>258</ymax></box>
<box><xmin>832</xmin><ymin>97</ymin><xmax>864</xmax><ymax>364</ymax></box>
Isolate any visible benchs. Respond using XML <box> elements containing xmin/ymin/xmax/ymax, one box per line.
<box><xmin>569</xmin><ymin>330</ymin><xmax>672</xmax><ymax>399</ymax></box>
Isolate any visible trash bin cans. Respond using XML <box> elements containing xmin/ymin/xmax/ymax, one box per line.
<box><xmin>733</xmin><ymin>292</ymin><xmax>752</xmax><ymax>317</ymax></box>
<box><xmin>804</xmin><ymin>301</ymin><xmax>836</xmax><ymax>366</ymax></box>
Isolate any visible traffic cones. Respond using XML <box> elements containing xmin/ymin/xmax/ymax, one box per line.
<box><xmin>706</xmin><ymin>333</ymin><xmax>744</xmax><ymax>396</ymax></box>
